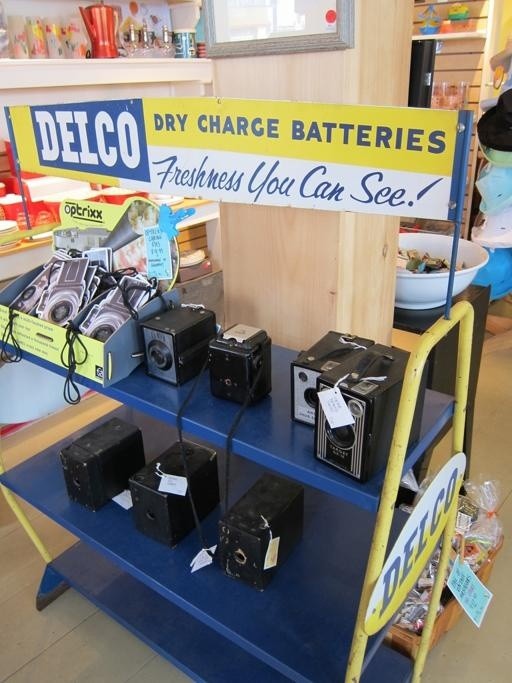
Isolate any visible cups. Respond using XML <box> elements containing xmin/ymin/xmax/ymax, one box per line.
<box><xmin>6</xmin><ymin>15</ymin><xmax>89</xmax><ymax>60</ymax></box>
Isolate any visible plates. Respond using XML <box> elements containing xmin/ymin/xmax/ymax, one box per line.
<box><xmin>0</xmin><ymin>219</ymin><xmax>20</xmax><ymax>251</ymax></box>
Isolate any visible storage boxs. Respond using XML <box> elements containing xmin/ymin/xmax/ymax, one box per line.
<box><xmin>384</xmin><ymin>534</ymin><xmax>504</xmax><ymax>660</ymax></box>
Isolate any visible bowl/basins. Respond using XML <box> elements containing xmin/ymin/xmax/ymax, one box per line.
<box><xmin>393</xmin><ymin>232</ymin><xmax>490</xmax><ymax>311</ymax></box>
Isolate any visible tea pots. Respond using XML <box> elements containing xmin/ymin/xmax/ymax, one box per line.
<box><xmin>78</xmin><ymin>1</ymin><xmax>123</xmax><ymax>59</ymax></box>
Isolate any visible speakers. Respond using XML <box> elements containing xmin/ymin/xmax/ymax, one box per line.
<box><xmin>142</xmin><ymin>304</ymin><xmax>215</xmax><ymax>386</ymax></box>
<box><xmin>128</xmin><ymin>441</ymin><xmax>220</xmax><ymax>546</ymax></box>
<box><xmin>290</xmin><ymin>331</ymin><xmax>376</xmax><ymax>428</ymax></box>
<box><xmin>210</xmin><ymin>325</ymin><xmax>271</xmax><ymax>405</ymax></box>
<box><xmin>60</xmin><ymin>418</ymin><xmax>145</xmax><ymax>511</ymax></box>
<box><xmin>316</xmin><ymin>343</ymin><xmax>430</xmax><ymax>483</ymax></box>
<box><xmin>219</xmin><ymin>474</ymin><xmax>304</xmax><ymax>591</ymax></box>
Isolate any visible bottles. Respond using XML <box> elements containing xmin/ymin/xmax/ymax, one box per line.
<box><xmin>127</xmin><ymin>24</ymin><xmax>174</xmax><ymax>57</ymax></box>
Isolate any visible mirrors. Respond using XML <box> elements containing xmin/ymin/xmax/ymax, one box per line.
<box><xmin>202</xmin><ymin>0</ymin><xmax>355</xmax><ymax>58</ymax></box>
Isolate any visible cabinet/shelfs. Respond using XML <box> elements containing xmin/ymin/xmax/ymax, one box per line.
<box><xmin>393</xmin><ymin>281</ymin><xmax>491</xmax><ymax>510</ymax></box>
<box><xmin>0</xmin><ymin>266</ymin><xmax>475</xmax><ymax>683</ymax></box>
<box><xmin>0</xmin><ymin>57</ymin><xmax>218</xmax><ymax>283</ymax></box>
